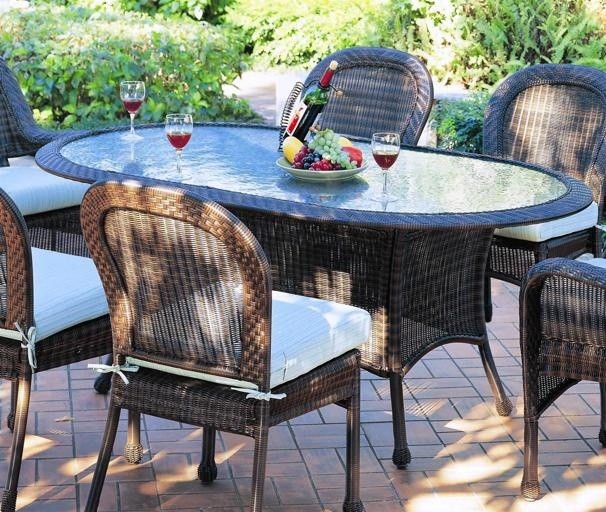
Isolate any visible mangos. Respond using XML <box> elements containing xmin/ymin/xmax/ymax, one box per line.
<box><xmin>282</xmin><ymin>136</ymin><xmax>304</xmax><ymax>165</ymax></box>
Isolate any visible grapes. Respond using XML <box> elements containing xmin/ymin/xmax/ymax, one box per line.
<box><xmin>292</xmin><ymin>130</ymin><xmax>358</xmax><ymax>171</ymax></box>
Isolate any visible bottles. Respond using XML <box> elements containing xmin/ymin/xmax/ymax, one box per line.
<box><xmin>281</xmin><ymin>61</ymin><xmax>337</xmax><ymax>149</ymax></box>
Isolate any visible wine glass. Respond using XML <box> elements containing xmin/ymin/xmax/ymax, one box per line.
<box><xmin>373</xmin><ymin>133</ymin><xmax>400</xmax><ymax>205</ymax></box>
<box><xmin>165</xmin><ymin>114</ymin><xmax>193</xmax><ymax>181</ymax></box>
<box><xmin>119</xmin><ymin>80</ymin><xmax>145</xmax><ymax>143</ymax></box>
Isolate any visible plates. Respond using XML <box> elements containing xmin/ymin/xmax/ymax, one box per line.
<box><xmin>277</xmin><ymin>156</ymin><xmax>368</xmax><ymax>180</ymax></box>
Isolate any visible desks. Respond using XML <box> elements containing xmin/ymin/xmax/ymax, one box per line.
<box><xmin>35</xmin><ymin>122</ymin><xmax>593</xmax><ymax>470</ymax></box>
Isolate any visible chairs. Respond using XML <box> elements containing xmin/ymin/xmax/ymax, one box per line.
<box><xmin>519</xmin><ymin>224</ymin><xmax>606</xmax><ymax>502</ymax></box>
<box><xmin>482</xmin><ymin>61</ymin><xmax>606</xmax><ymax>322</ymax></box>
<box><xmin>0</xmin><ymin>188</ymin><xmax>144</xmax><ymax>512</ymax></box>
<box><xmin>0</xmin><ymin>58</ymin><xmax>89</xmax><ymax>256</ymax></box>
<box><xmin>78</xmin><ymin>177</ymin><xmax>372</xmax><ymax>512</ymax></box>
<box><xmin>300</xmin><ymin>46</ymin><xmax>434</xmax><ymax>145</ymax></box>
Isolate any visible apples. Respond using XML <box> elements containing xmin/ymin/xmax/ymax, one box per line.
<box><xmin>342</xmin><ymin>146</ymin><xmax>362</xmax><ymax>168</ymax></box>
